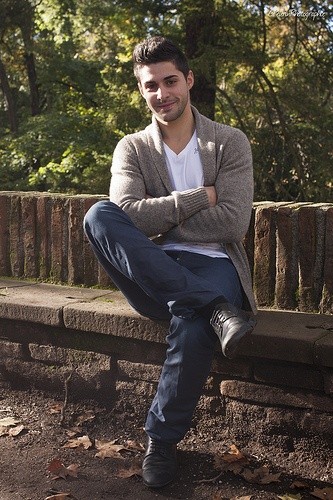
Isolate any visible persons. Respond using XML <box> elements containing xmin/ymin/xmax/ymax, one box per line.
<box><xmin>82</xmin><ymin>35</ymin><xmax>259</xmax><ymax>488</ymax></box>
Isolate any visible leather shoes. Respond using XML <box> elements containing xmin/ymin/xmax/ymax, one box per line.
<box><xmin>208</xmin><ymin>307</ymin><xmax>256</xmax><ymax>361</ymax></box>
<box><xmin>140</xmin><ymin>437</ymin><xmax>180</xmax><ymax>488</ymax></box>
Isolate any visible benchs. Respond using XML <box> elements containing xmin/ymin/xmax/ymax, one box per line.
<box><xmin>0</xmin><ymin>190</ymin><xmax>333</xmax><ymax>465</ymax></box>
